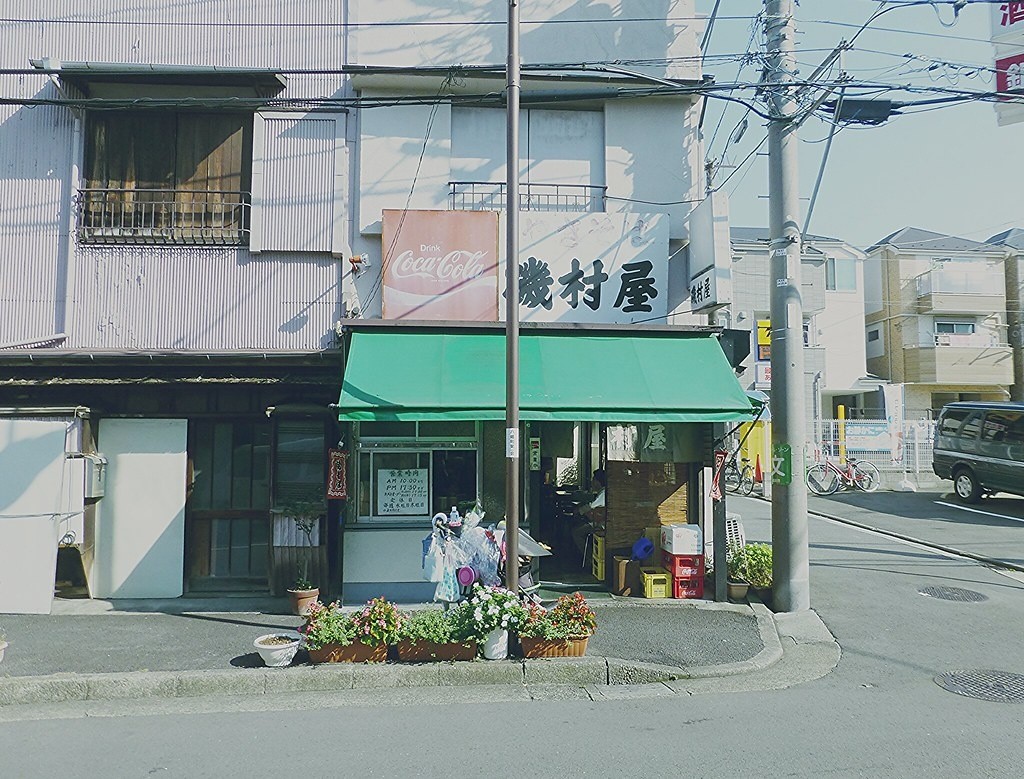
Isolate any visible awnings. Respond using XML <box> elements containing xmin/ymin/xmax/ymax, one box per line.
<box><xmin>328</xmin><ymin>331</ymin><xmax>758</xmax><ymax>423</ymax></box>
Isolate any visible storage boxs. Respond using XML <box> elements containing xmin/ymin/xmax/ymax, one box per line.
<box><xmin>659</xmin><ymin>524</ymin><xmax>704</xmax><ymax>555</ymax></box>
<box><xmin>673</xmin><ymin>576</ymin><xmax>704</xmax><ymax>598</ymax></box>
<box><xmin>591</xmin><ymin>554</ymin><xmax>605</xmax><ymax>581</ymax></box>
<box><xmin>661</xmin><ymin>549</ymin><xmax>705</xmax><ymax>576</ymax></box>
<box><xmin>639</xmin><ymin>566</ymin><xmax>673</xmax><ymax>599</ymax></box>
<box><xmin>592</xmin><ymin>531</ymin><xmax>605</xmax><ymax>560</ymax></box>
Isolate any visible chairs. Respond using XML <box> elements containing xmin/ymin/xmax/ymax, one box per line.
<box><xmin>582</xmin><ymin>506</ymin><xmax>607</xmax><ymax>567</ymax></box>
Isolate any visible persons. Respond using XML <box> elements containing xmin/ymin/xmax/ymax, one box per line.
<box><xmin>570</xmin><ymin>469</ymin><xmax>605</xmax><ymax>568</ymax></box>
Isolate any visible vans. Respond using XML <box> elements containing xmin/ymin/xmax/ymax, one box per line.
<box><xmin>932</xmin><ymin>400</ymin><xmax>1024</xmax><ymax>504</ymax></box>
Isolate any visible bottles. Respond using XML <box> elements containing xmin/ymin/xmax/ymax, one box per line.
<box><xmin>450</xmin><ymin>506</ymin><xmax>459</xmax><ymax>522</ymax></box>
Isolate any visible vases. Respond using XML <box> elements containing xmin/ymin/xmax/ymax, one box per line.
<box><xmin>396</xmin><ymin>638</ymin><xmax>478</xmax><ymax>662</ymax></box>
<box><xmin>482</xmin><ymin>625</ymin><xmax>509</xmax><ymax>659</ymax></box>
<box><xmin>521</xmin><ymin>636</ymin><xmax>590</xmax><ymax>658</ymax></box>
<box><xmin>307</xmin><ymin>636</ymin><xmax>389</xmax><ymax>663</ymax></box>
<box><xmin>253</xmin><ymin>633</ymin><xmax>302</xmax><ymax>666</ymax></box>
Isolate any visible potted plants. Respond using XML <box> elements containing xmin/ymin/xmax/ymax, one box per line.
<box><xmin>285</xmin><ymin>488</ymin><xmax>329</xmax><ymax>617</ymax></box>
<box><xmin>726</xmin><ymin>542</ymin><xmax>773</xmax><ymax>600</ymax></box>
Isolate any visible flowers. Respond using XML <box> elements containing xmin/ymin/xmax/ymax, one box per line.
<box><xmin>460</xmin><ymin>581</ymin><xmax>523</xmax><ymax>633</ymax></box>
<box><xmin>517</xmin><ymin>590</ymin><xmax>598</xmax><ymax>648</ymax></box>
<box><xmin>297</xmin><ymin>596</ymin><xmax>410</xmax><ymax>651</ymax></box>
<box><xmin>399</xmin><ymin>603</ymin><xmax>471</xmax><ymax>644</ymax></box>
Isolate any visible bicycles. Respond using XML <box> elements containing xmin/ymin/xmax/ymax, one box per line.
<box><xmin>724</xmin><ymin>444</ymin><xmax>756</xmax><ymax>495</ymax></box>
<box><xmin>806</xmin><ymin>443</ymin><xmax>881</xmax><ymax>496</ymax></box>
